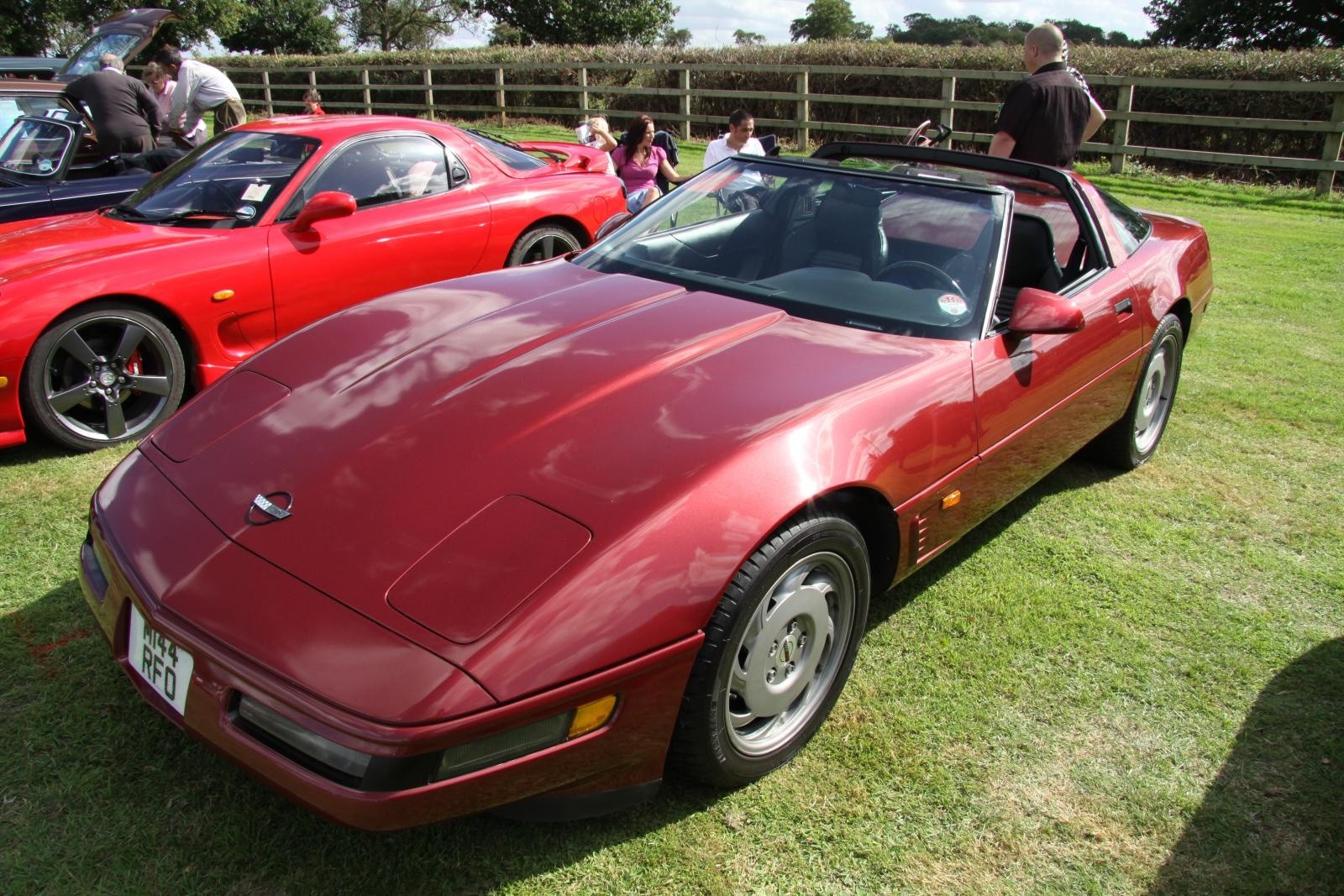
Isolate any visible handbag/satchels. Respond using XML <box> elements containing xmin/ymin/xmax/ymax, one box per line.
<box><xmin>575</xmin><ymin>124</ymin><xmax>597</xmax><ymax>149</ymax></box>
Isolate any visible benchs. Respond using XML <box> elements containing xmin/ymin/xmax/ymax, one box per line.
<box><xmin>102</xmin><ymin>149</ymin><xmax>189</xmax><ymax>178</ymax></box>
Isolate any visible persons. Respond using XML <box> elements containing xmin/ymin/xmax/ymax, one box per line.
<box><xmin>139</xmin><ymin>59</ymin><xmax>207</xmax><ymax>153</ymax></box>
<box><xmin>292</xmin><ymin>89</ymin><xmax>329</xmax><ymax>155</ymax></box>
<box><xmin>984</xmin><ymin>23</ymin><xmax>1107</xmax><ymax>187</ymax></box>
<box><xmin>701</xmin><ymin>107</ymin><xmax>780</xmax><ymax>219</ymax></box>
<box><xmin>581</xmin><ymin>116</ymin><xmax>617</xmax><ymax>178</ymax></box>
<box><xmin>610</xmin><ymin>114</ymin><xmax>695</xmax><ymax>224</ymax></box>
<box><xmin>152</xmin><ymin>42</ymin><xmax>249</xmax><ymax>142</ymax></box>
<box><xmin>65</xmin><ymin>55</ymin><xmax>163</xmax><ymax>161</ymax></box>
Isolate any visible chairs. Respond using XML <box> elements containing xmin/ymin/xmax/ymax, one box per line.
<box><xmin>936</xmin><ymin>212</ymin><xmax>1063</xmax><ymax>333</ymax></box>
<box><xmin>707</xmin><ymin>134</ymin><xmax>777</xmax><ymax>218</ymax></box>
<box><xmin>780</xmin><ymin>182</ymin><xmax>888</xmax><ymax>278</ymax></box>
<box><xmin>617</xmin><ymin>131</ymin><xmax>682</xmax><ymax>229</ymax></box>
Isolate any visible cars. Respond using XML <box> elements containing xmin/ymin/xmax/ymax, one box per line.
<box><xmin>0</xmin><ymin>10</ymin><xmax>308</xmax><ymax>224</ymax></box>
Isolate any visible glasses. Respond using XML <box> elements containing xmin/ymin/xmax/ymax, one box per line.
<box><xmin>741</xmin><ymin>127</ymin><xmax>753</xmax><ymax>132</ymax></box>
<box><xmin>146</xmin><ymin>77</ymin><xmax>162</xmax><ymax>84</ymax></box>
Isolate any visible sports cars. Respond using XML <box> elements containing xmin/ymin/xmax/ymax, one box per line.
<box><xmin>0</xmin><ymin>113</ymin><xmax>638</xmax><ymax>459</ymax></box>
<box><xmin>76</xmin><ymin>134</ymin><xmax>1216</xmax><ymax>838</ymax></box>
